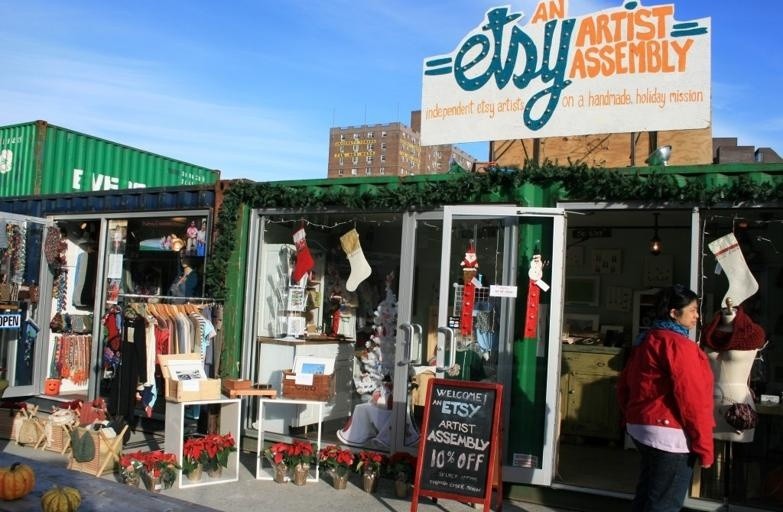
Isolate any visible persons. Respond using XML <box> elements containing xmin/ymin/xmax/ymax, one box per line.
<box><xmin>697</xmin><ymin>297</ymin><xmax>765</xmax><ymax>444</ymax></box>
<box><xmin>161</xmin><ymin>247</ymin><xmax>202</xmax><ymax>304</ymax></box>
<box><xmin>185</xmin><ymin>219</ymin><xmax>198</xmax><ymax>256</ymax></box>
<box><xmin>614</xmin><ymin>283</ymin><xmax>717</xmax><ymax>512</ymax></box>
<box><xmin>196</xmin><ymin>221</ymin><xmax>206</xmax><ymax>256</ymax></box>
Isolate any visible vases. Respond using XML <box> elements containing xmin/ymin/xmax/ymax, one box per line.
<box><xmin>360</xmin><ymin>472</ymin><xmax>377</xmax><ymax>493</ymax></box>
<box><xmin>391</xmin><ymin>471</ymin><xmax>410</xmax><ymax>499</ymax></box>
<box><xmin>270</xmin><ymin>463</ymin><xmax>289</xmax><ymax>483</ymax></box>
<box><xmin>327</xmin><ymin>467</ymin><xmax>350</xmax><ymax>489</ymax></box>
<box><xmin>292</xmin><ymin>465</ymin><xmax>308</xmax><ymax>486</ymax></box>
<box><xmin>137</xmin><ymin>466</ymin><xmax>162</xmax><ymax>493</ymax></box>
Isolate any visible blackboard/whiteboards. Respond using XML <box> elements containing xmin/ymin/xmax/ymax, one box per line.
<box><xmin>414</xmin><ymin>378</ymin><xmax>504</xmax><ymax>504</ymax></box>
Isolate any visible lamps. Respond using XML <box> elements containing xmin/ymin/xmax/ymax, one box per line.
<box><xmin>648</xmin><ymin>212</ymin><xmax>664</xmax><ymax>256</ymax></box>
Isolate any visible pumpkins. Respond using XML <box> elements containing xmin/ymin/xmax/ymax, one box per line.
<box><xmin>0</xmin><ymin>462</ymin><xmax>35</xmax><ymax>500</ymax></box>
<box><xmin>41</xmin><ymin>485</ymin><xmax>81</xmax><ymax>512</ymax></box>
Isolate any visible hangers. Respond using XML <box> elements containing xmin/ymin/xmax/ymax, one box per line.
<box><xmin>117</xmin><ymin>293</ymin><xmax>215</xmax><ymax>328</ymax></box>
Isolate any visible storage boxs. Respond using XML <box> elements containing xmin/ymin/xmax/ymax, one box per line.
<box><xmin>281</xmin><ymin>365</ymin><xmax>337</xmax><ymax>404</ymax></box>
<box><xmin>156</xmin><ymin>352</ymin><xmax>221</xmax><ymax>404</ymax></box>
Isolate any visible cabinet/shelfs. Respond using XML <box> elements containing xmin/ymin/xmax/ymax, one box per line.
<box><xmin>556</xmin><ymin>342</ymin><xmax>626</xmax><ymax>448</ymax></box>
<box><xmin>253</xmin><ymin>331</ymin><xmax>357</xmax><ymax>436</ymax></box>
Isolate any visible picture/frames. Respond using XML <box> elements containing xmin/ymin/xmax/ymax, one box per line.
<box><xmin>564</xmin><ymin>275</ymin><xmax>601</xmax><ymax>308</ymax></box>
<box><xmin>642</xmin><ymin>255</ymin><xmax>675</xmax><ymax>289</ymax></box>
<box><xmin>563</xmin><ymin>313</ymin><xmax>600</xmax><ymax>335</ymax></box>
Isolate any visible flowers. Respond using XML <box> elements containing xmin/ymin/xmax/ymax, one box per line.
<box><xmin>261</xmin><ymin>438</ymin><xmax>419</xmax><ymax>480</ymax></box>
<box><xmin>111</xmin><ymin>432</ymin><xmax>239</xmax><ymax>488</ymax></box>
